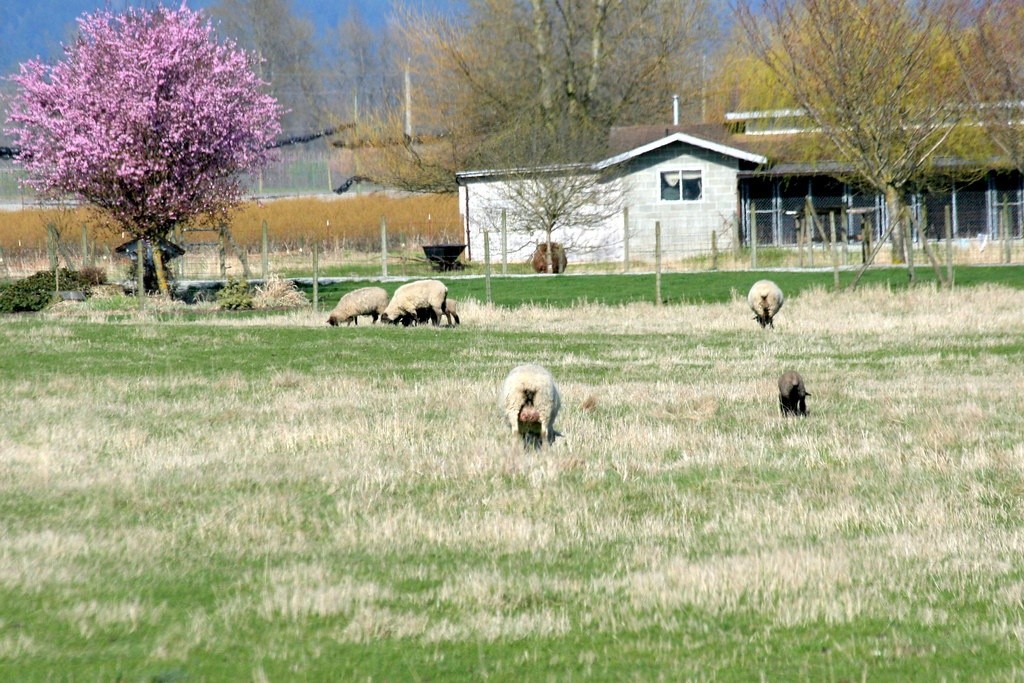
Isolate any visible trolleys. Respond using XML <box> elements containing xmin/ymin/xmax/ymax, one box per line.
<box><xmin>411</xmin><ymin>244</ymin><xmax>468</xmax><ymax>272</ymax></box>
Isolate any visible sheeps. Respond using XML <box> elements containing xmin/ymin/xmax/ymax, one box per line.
<box><xmin>325</xmin><ymin>287</ymin><xmax>389</xmax><ymax>328</ymax></box>
<box><xmin>381</xmin><ymin>279</ymin><xmax>460</xmax><ymax>328</ymax></box>
<box><xmin>779</xmin><ymin>370</ymin><xmax>812</xmax><ymax>417</ymax></box>
<box><xmin>747</xmin><ymin>279</ymin><xmax>785</xmax><ymax>328</ymax></box>
<box><xmin>500</xmin><ymin>363</ymin><xmax>560</xmax><ymax>452</ymax></box>
